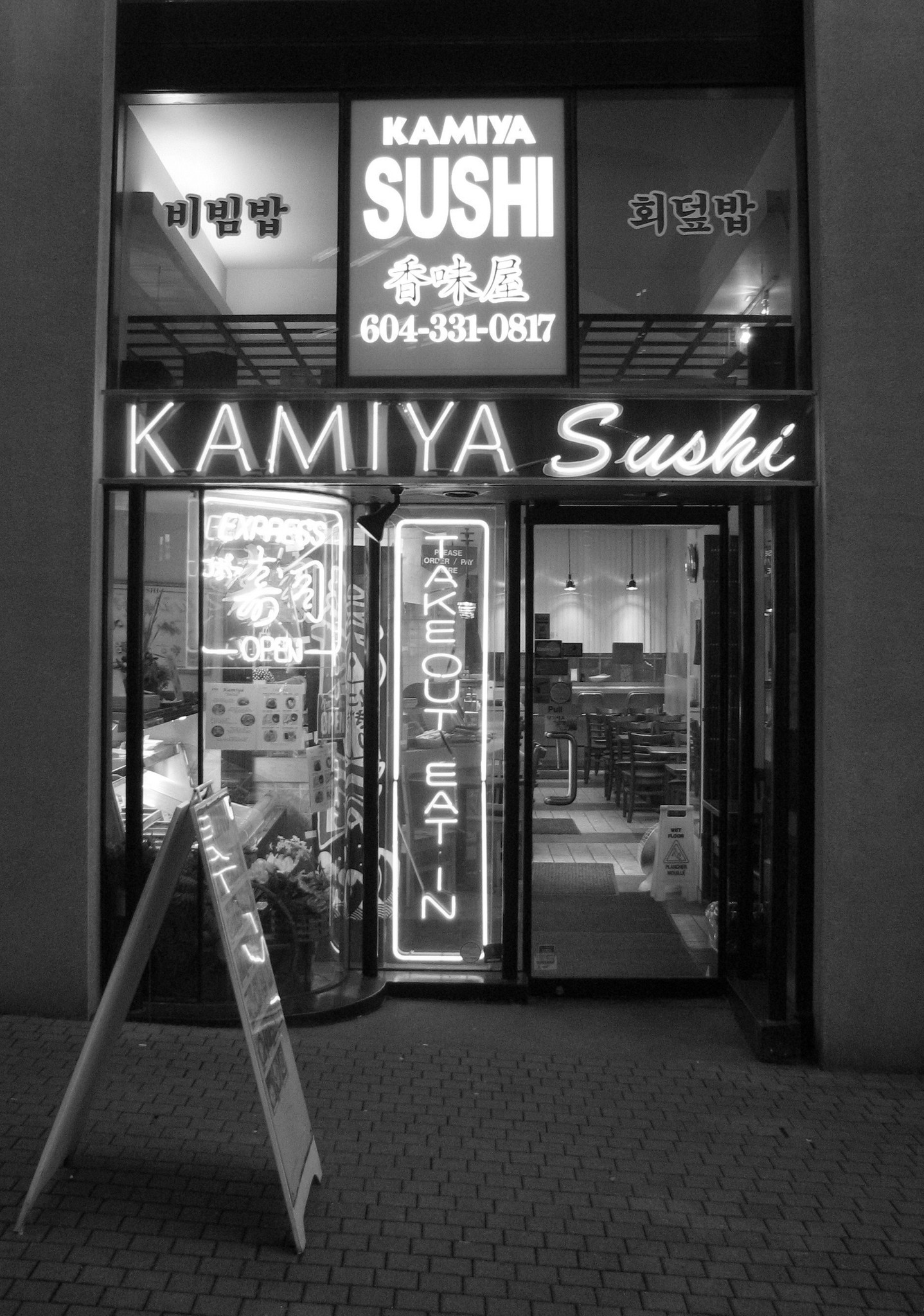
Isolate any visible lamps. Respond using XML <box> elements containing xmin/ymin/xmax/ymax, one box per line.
<box><xmin>564</xmin><ymin>526</ymin><xmax>577</xmax><ymax>591</ymax></box>
<box><xmin>626</xmin><ymin>529</ymin><xmax>640</xmax><ymax>592</ymax></box>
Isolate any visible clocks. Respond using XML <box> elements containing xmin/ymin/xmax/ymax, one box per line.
<box><xmin>683</xmin><ymin>543</ymin><xmax>698</xmax><ymax>584</ymax></box>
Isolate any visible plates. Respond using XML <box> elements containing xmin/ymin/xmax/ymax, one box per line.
<box><xmin>211</xmin><ymin>697</ymin><xmax>299</xmax><ymax>743</ymax></box>
<box><xmin>314</xmin><ymin>757</ymin><xmax>335</xmax><ymax>803</ymax></box>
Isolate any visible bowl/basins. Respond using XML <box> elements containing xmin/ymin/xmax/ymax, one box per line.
<box><xmin>588</xmin><ymin>674</ymin><xmax>610</xmax><ymax>682</ymax></box>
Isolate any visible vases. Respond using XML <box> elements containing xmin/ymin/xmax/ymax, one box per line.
<box><xmin>112</xmin><ymin>689</ymin><xmax>176</xmax><ymax>712</ymax></box>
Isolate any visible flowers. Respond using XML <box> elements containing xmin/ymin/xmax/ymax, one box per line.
<box><xmin>111</xmin><ymin>589</ymin><xmax>184</xmax><ymax>695</ymax></box>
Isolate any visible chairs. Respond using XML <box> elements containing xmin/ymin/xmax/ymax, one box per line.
<box><xmin>582</xmin><ymin>710</ymin><xmax>694</xmax><ymax>823</ymax></box>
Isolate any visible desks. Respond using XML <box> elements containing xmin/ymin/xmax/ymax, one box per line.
<box><xmin>664</xmin><ymin>762</ymin><xmax>687</xmax><ymax>805</ymax></box>
<box><xmin>648</xmin><ymin>747</ymin><xmax>687</xmax><ymax>785</ymax></box>
